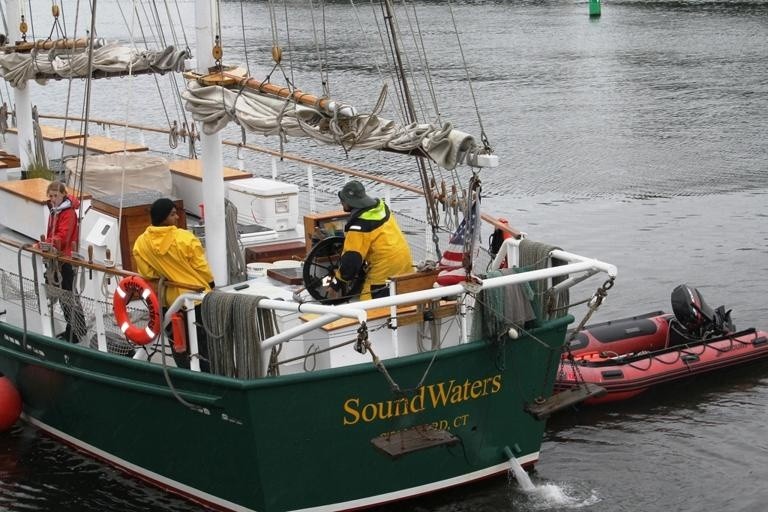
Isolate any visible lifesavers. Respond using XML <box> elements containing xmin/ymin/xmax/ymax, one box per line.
<box><xmin>113</xmin><ymin>276</ymin><xmax>162</xmax><ymax>344</ymax></box>
<box><xmin>494</xmin><ymin>217</ymin><xmax>512</xmax><ymax>270</ymax></box>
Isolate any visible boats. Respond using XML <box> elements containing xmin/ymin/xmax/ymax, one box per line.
<box><xmin>544</xmin><ymin>356</ymin><xmax>767</xmax><ymax>430</ymax></box>
<box><xmin>553</xmin><ymin>283</ymin><xmax>767</xmax><ymax>404</ymax></box>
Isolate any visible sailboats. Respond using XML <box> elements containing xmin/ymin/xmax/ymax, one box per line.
<box><xmin>0</xmin><ymin>0</ymin><xmax>619</xmax><ymax>512</ymax></box>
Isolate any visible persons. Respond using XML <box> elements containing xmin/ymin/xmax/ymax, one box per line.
<box><xmin>133</xmin><ymin>198</ymin><xmax>216</xmax><ymax>373</ymax></box>
<box><xmin>326</xmin><ymin>181</ymin><xmax>416</xmax><ymax>300</ymax></box>
<box><xmin>34</xmin><ymin>181</ymin><xmax>86</xmax><ymax>342</ymax></box>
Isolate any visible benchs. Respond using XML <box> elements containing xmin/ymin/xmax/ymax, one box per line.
<box><xmin>296</xmin><ymin>300</ymin><xmax>460</xmax><ymax>371</ymax></box>
<box><xmin>1</xmin><ymin>175</ymin><xmax>92</xmax><ymax>242</ymax></box>
<box><xmin>59</xmin><ymin>136</ymin><xmax>150</xmax><ymax>168</ymax></box>
<box><xmin>171</xmin><ymin>157</ymin><xmax>252</xmax><ymax>222</ymax></box>
<box><xmin>5</xmin><ymin>122</ymin><xmax>84</xmax><ymax>166</ymax></box>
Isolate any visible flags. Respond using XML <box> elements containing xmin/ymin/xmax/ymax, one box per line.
<box><xmin>438</xmin><ymin>198</ymin><xmax>475</xmax><ymax>284</ymax></box>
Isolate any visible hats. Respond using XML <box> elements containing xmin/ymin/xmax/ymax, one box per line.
<box><xmin>338</xmin><ymin>180</ymin><xmax>377</xmax><ymax>209</ymax></box>
<box><xmin>150</xmin><ymin>198</ymin><xmax>177</xmax><ymax>227</ymax></box>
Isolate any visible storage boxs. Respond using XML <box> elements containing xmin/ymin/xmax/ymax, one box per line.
<box><xmin>226</xmin><ymin>175</ymin><xmax>299</xmax><ymax>231</ymax></box>
